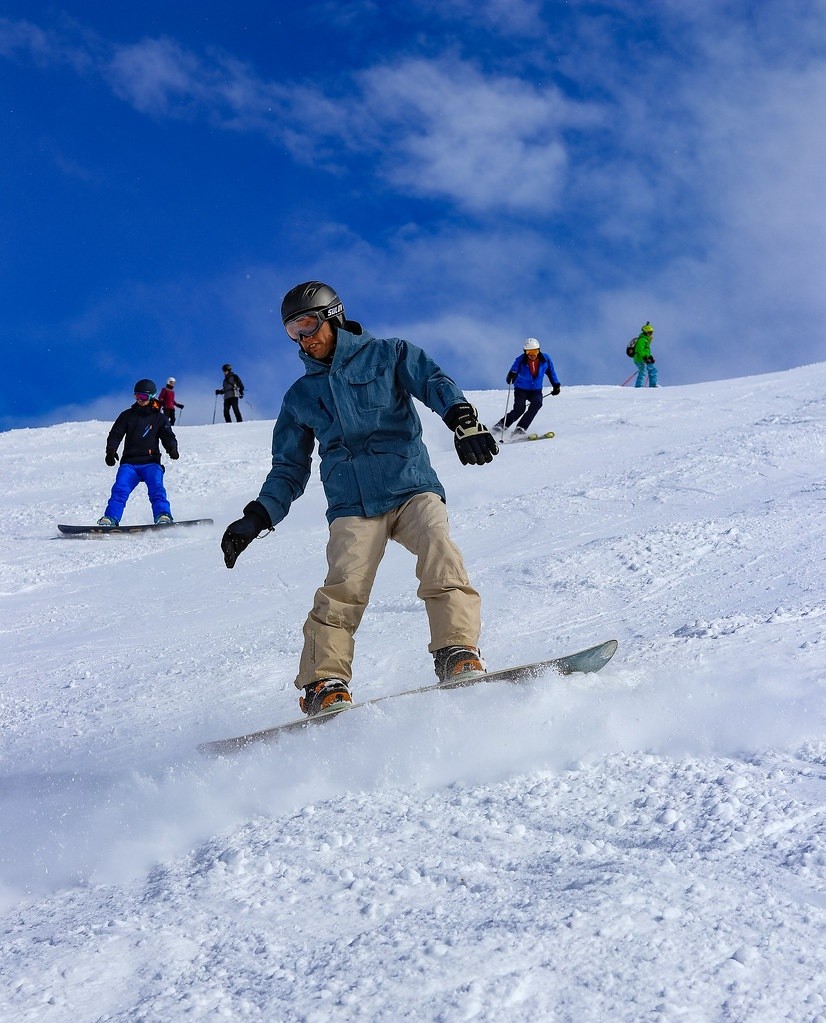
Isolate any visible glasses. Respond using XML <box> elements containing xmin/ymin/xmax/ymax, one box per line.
<box><xmin>223</xmin><ymin>369</ymin><xmax>228</xmax><ymax>372</ymax></box>
<box><xmin>134</xmin><ymin>392</ymin><xmax>153</xmax><ymax>401</ymax></box>
<box><xmin>284</xmin><ymin>301</ymin><xmax>345</xmax><ymax>343</ymax></box>
<box><xmin>524</xmin><ymin>349</ymin><xmax>539</xmax><ymax>355</ymax></box>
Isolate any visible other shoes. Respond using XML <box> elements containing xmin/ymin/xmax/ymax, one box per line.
<box><xmin>97</xmin><ymin>516</ymin><xmax>119</xmax><ymax>525</ymax></box>
<box><xmin>512</xmin><ymin>426</ymin><xmax>526</xmax><ymax>435</ymax></box>
<box><xmin>156</xmin><ymin>514</ymin><xmax>173</xmax><ymax>524</ymax></box>
<box><xmin>493</xmin><ymin>421</ymin><xmax>507</xmax><ymax>431</ymax></box>
<box><xmin>649</xmin><ymin>384</ymin><xmax>661</xmax><ymax>388</ymax></box>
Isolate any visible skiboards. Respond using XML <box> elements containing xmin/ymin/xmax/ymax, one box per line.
<box><xmin>496</xmin><ymin>432</ymin><xmax>555</xmax><ymax>447</ymax></box>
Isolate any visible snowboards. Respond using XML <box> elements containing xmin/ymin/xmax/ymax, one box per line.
<box><xmin>198</xmin><ymin>636</ymin><xmax>620</xmax><ymax>758</ymax></box>
<box><xmin>56</xmin><ymin>517</ymin><xmax>215</xmax><ymax>536</ymax></box>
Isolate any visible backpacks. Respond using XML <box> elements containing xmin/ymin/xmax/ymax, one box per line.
<box><xmin>626</xmin><ymin>336</ymin><xmax>639</xmax><ymax>357</ymax></box>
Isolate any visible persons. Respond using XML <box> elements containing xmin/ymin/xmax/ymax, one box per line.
<box><xmin>492</xmin><ymin>337</ymin><xmax>561</xmax><ymax>436</ymax></box>
<box><xmin>97</xmin><ymin>379</ymin><xmax>180</xmax><ymax>527</ymax></box>
<box><xmin>216</xmin><ymin>363</ymin><xmax>244</xmax><ymax>423</ymax></box>
<box><xmin>221</xmin><ymin>281</ymin><xmax>500</xmax><ymax>721</ymax></box>
<box><xmin>158</xmin><ymin>377</ymin><xmax>184</xmax><ymax>426</ymax></box>
<box><xmin>633</xmin><ymin>324</ymin><xmax>660</xmax><ymax>387</ymax></box>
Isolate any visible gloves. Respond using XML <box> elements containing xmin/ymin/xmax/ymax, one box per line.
<box><xmin>642</xmin><ymin>355</ymin><xmax>650</xmax><ymax>365</ymax></box>
<box><xmin>507</xmin><ymin>371</ymin><xmax>517</xmax><ymax>383</ymax></box>
<box><xmin>648</xmin><ymin>355</ymin><xmax>655</xmax><ymax>364</ymax></box>
<box><xmin>176</xmin><ymin>404</ymin><xmax>184</xmax><ymax>409</ymax></box>
<box><xmin>551</xmin><ymin>383</ymin><xmax>561</xmax><ymax>395</ymax></box>
<box><xmin>443</xmin><ymin>403</ymin><xmax>499</xmax><ymax>465</ymax></box>
<box><xmin>239</xmin><ymin>391</ymin><xmax>244</xmax><ymax>398</ymax></box>
<box><xmin>105</xmin><ymin>447</ymin><xmax>119</xmax><ymax>466</ymax></box>
<box><xmin>221</xmin><ymin>500</ymin><xmax>275</xmax><ymax>568</ymax></box>
<box><xmin>216</xmin><ymin>390</ymin><xmax>223</xmax><ymax>395</ymax></box>
<box><xmin>166</xmin><ymin>447</ymin><xmax>179</xmax><ymax>460</ymax></box>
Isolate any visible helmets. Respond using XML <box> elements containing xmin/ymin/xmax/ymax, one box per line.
<box><xmin>641</xmin><ymin>325</ymin><xmax>654</xmax><ymax>332</ymax></box>
<box><xmin>167</xmin><ymin>377</ymin><xmax>176</xmax><ymax>386</ymax></box>
<box><xmin>523</xmin><ymin>337</ymin><xmax>540</xmax><ymax>350</ymax></box>
<box><xmin>134</xmin><ymin>379</ymin><xmax>157</xmax><ymax>395</ymax></box>
<box><xmin>222</xmin><ymin>364</ymin><xmax>232</xmax><ymax>370</ymax></box>
<box><xmin>281</xmin><ymin>281</ymin><xmax>346</xmax><ymax>329</ymax></box>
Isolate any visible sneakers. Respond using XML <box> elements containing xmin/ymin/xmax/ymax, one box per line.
<box><xmin>432</xmin><ymin>645</ymin><xmax>488</xmax><ymax>683</ymax></box>
<box><xmin>299</xmin><ymin>677</ymin><xmax>353</xmax><ymax>717</ymax></box>
